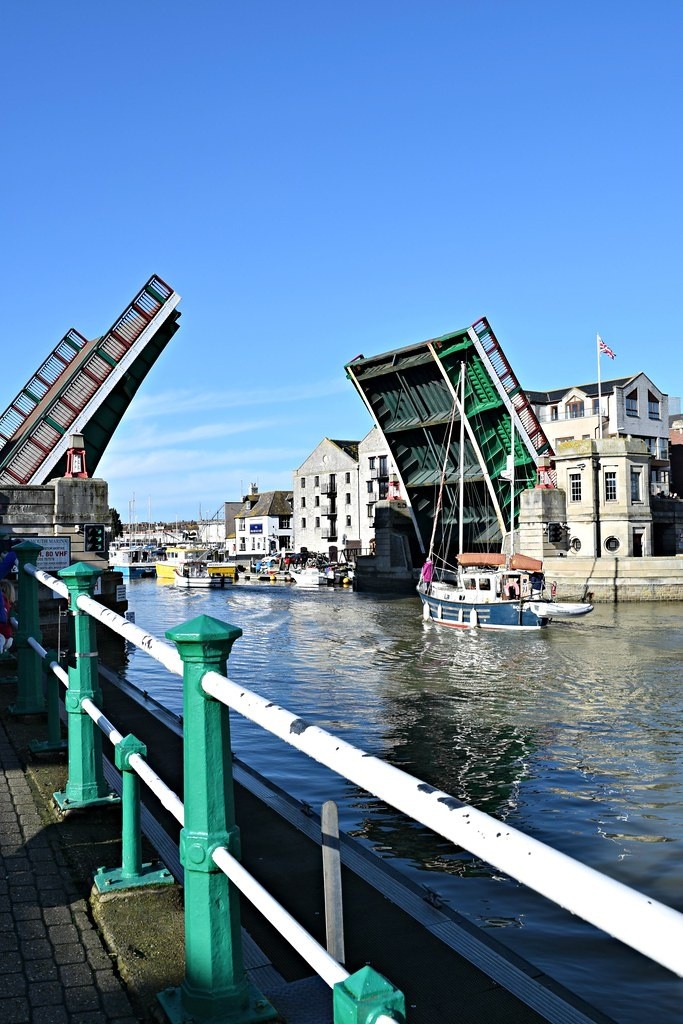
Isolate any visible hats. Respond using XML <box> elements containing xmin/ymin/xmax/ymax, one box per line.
<box><xmin>426</xmin><ymin>558</ymin><xmax>430</xmax><ymax>561</ymax></box>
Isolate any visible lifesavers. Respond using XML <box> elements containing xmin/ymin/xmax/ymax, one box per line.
<box><xmin>506</xmin><ymin>582</ymin><xmax>520</xmax><ymax>597</ymax></box>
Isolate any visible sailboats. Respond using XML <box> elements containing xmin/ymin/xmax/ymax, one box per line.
<box><xmin>417</xmin><ymin>360</ymin><xmax>593</xmax><ymax>631</ymax></box>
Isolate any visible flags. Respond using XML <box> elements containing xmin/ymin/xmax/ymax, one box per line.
<box><xmin>599</xmin><ymin>337</ymin><xmax>616</xmax><ymax>360</ymax></box>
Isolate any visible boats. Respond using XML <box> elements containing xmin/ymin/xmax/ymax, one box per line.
<box><xmin>109</xmin><ymin>491</ymin><xmax>339</xmax><ymax>594</ymax></box>
<box><xmin>520</xmin><ymin>600</ymin><xmax>593</xmax><ymax>617</ymax></box>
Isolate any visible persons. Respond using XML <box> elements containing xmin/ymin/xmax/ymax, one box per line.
<box><xmin>421</xmin><ymin>557</ymin><xmax>434</xmax><ymax>594</ymax></box>
<box><xmin>552</xmin><ymin>581</ymin><xmax>557</xmax><ymax>602</ymax></box>
<box><xmin>273</xmin><ymin>547</ymin><xmax>277</xmax><ymax>553</ymax></box>
<box><xmin>232</xmin><ymin>544</ymin><xmax>236</xmax><ymax>552</ymax></box>
<box><xmin>0</xmin><ymin>580</ymin><xmax>16</xmax><ymax>654</ymax></box>
